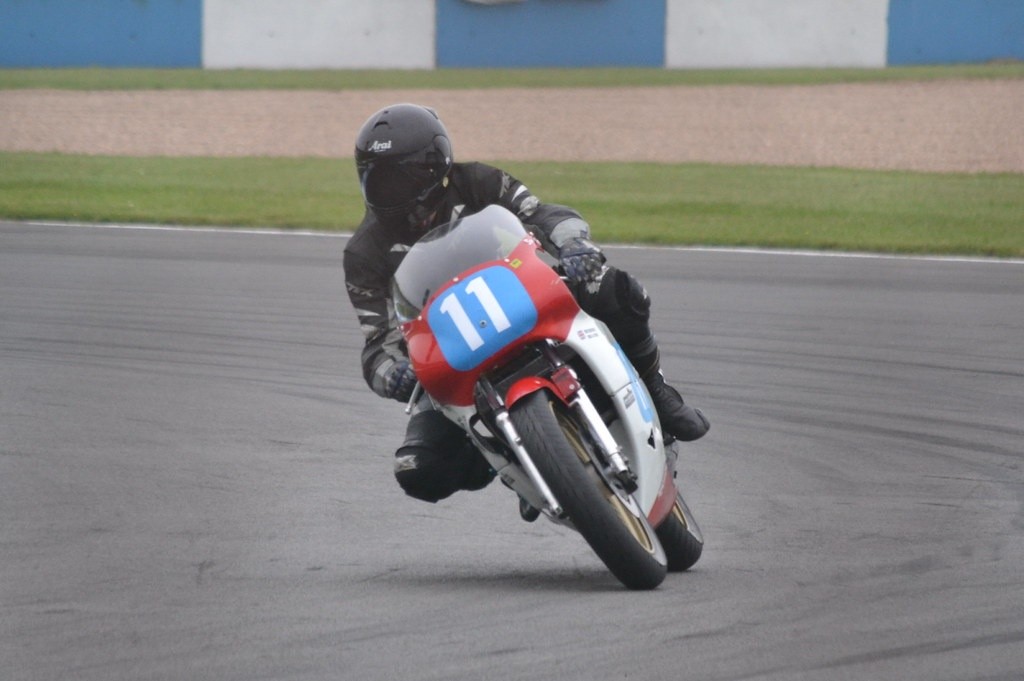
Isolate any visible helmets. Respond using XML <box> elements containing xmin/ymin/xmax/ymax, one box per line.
<box><xmin>354</xmin><ymin>103</ymin><xmax>453</xmax><ymax>230</ymax></box>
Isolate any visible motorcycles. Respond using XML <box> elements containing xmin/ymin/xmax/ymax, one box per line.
<box><xmin>389</xmin><ymin>203</ymin><xmax>704</xmax><ymax>590</ymax></box>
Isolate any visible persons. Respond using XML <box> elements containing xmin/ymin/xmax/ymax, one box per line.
<box><xmin>342</xmin><ymin>105</ymin><xmax>713</xmax><ymax>523</ymax></box>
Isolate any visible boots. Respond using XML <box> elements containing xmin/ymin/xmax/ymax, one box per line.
<box><xmin>624</xmin><ymin>328</ymin><xmax>710</xmax><ymax>441</ymax></box>
<box><xmin>519</xmin><ymin>497</ymin><xmax>540</xmax><ymax>522</ymax></box>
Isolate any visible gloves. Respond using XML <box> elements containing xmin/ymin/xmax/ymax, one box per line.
<box><xmin>371</xmin><ymin>355</ymin><xmax>424</xmax><ymax>403</ymax></box>
<box><xmin>560</xmin><ymin>237</ymin><xmax>608</xmax><ymax>288</ymax></box>
